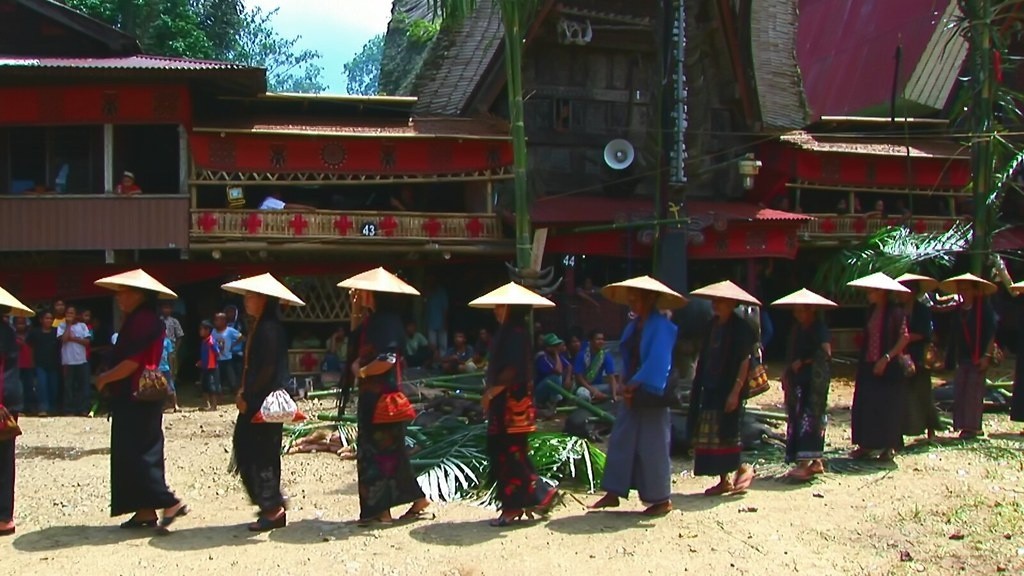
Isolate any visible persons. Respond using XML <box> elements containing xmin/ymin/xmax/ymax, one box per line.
<box><xmin>335</xmin><ymin>265</ymin><xmax>434</xmax><ymax>528</ymax></box>
<box><xmin>686</xmin><ymin>280</ymin><xmax>769</xmax><ymax>495</ymax></box>
<box><xmin>322</xmin><ymin>313</ymin><xmax>650</xmax><ymax>417</ymax></box>
<box><xmin>938</xmin><ymin>271</ymin><xmax>999</xmax><ymax>438</ymax></box>
<box><xmin>291</xmin><ymin>325</ymin><xmax>319</xmax><ymax>350</ymax></box>
<box><xmin>220</xmin><ymin>272</ymin><xmax>306</xmax><ymax>532</ymax></box>
<box><xmin>768</xmin><ymin>287</ymin><xmax>838</xmax><ymax>480</ymax></box>
<box><xmin>845</xmin><ymin>271</ymin><xmax>941</xmax><ymax>463</ymax></box>
<box><xmin>94</xmin><ymin>268</ymin><xmax>191</xmax><ymax>532</ymax></box>
<box><xmin>468</xmin><ymin>281</ymin><xmax>560</xmax><ymax>526</ymax></box>
<box><xmin>255</xmin><ymin>179</ymin><xmax>489</xmax><ymax>216</ymax></box>
<box><xmin>0</xmin><ymin>292</ymin><xmax>250</xmax><ymax>416</ymax></box>
<box><xmin>584</xmin><ymin>275</ymin><xmax>688</xmax><ymax>516</ymax></box>
<box><xmin>0</xmin><ymin>284</ymin><xmax>34</xmax><ymax>536</ymax></box>
<box><xmin>19</xmin><ymin>180</ymin><xmax>57</xmax><ymax>194</ymax></box>
<box><xmin>1000</xmin><ymin>273</ymin><xmax>1024</xmax><ymax>435</ymax></box>
<box><xmin>115</xmin><ymin>173</ymin><xmax>140</xmax><ymax>193</ymax></box>
<box><xmin>791</xmin><ymin>186</ymin><xmax>977</xmax><ymax>230</ymax></box>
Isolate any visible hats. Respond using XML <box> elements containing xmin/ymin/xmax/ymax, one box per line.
<box><xmin>691</xmin><ymin>279</ymin><xmax>761</xmax><ymax>307</ymax></box>
<box><xmin>846</xmin><ymin>272</ymin><xmax>911</xmax><ymax>295</ymax></box>
<box><xmin>600</xmin><ymin>276</ymin><xmax>688</xmax><ymax>309</ymax></box>
<box><xmin>770</xmin><ymin>287</ymin><xmax>837</xmax><ymax>310</ymax></box>
<box><xmin>543</xmin><ymin>333</ymin><xmax>563</xmax><ymax>345</ymax></box>
<box><xmin>0</xmin><ymin>284</ymin><xmax>35</xmax><ymax>318</ymax></box>
<box><xmin>93</xmin><ymin>267</ymin><xmax>179</xmax><ymax>300</ymax></box>
<box><xmin>468</xmin><ymin>282</ymin><xmax>557</xmax><ymax>308</ymax></box>
<box><xmin>895</xmin><ymin>274</ymin><xmax>936</xmax><ymax>292</ymax></box>
<box><xmin>939</xmin><ymin>273</ymin><xmax>997</xmax><ymax>295</ymax></box>
<box><xmin>221</xmin><ymin>271</ymin><xmax>305</xmax><ymax>308</ymax></box>
<box><xmin>337</xmin><ymin>266</ymin><xmax>421</xmax><ymax>295</ymax></box>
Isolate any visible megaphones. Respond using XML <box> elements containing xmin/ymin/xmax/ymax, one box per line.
<box><xmin>604</xmin><ymin>138</ymin><xmax>635</xmax><ymax>169</ymax></box>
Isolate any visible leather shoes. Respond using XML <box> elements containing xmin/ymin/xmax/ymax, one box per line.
<box><xmin>119</xmin><ymin>504</ymin><xmax>187</xmax><ymax>528</ymax></box>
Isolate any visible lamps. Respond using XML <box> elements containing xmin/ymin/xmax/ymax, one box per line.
<box><xmin>227</xmin><ymin>186</ymin><xmax>246</xmax><ymax>208</ymax></box>
<box><xmin>738</xmin><ymin>154</ymin><xmax>762</xmax><ymax>189</ymax></box>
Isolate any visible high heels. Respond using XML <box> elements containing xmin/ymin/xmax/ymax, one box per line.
<box><xmin>489</xmin><ymin>491</ymin><xmax>565</xmax><ymax>527</ymax></box>
<box><xmin>247</xmin><ymin>502</ymin><xmax>287</xmax><ymax>531</ymax></box>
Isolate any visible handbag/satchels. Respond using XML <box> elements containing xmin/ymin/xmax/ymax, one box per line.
<box><xmin>899</xmin><ymin>353</ymin><xmax>916</xmax><ymax>377</ymax></box>
<box><xmin>140</xmin><ymin>364</ymin><xmax>168</xmax><ymax>402</ymax></box>
<box><xmin>639</xmin><ymin>382</ymin><xmax>676</xmax><ymax>407</ymax></box>
<box><xmin>371</xmin><ymin>392</ymin><xmax>417</xmax><ymax>423</ymax></box>
<box><xmin>261</xmin><ymin>387</ymin><xmax>297</xmax><ymax>422</ymax></box>
<box><xmin>734</xmin><ymin>355</ymin><xmax>770</xmax><ymax>401</ymax></box>
<box><xmin>504</xmin><ymin>394</ymin><xmax>537</xmax><ymax>433</ymax></box>
<box><xmin>0</xmin><ymin>404</ymin><xmax>22</xmax><ymax>441</ymax></box>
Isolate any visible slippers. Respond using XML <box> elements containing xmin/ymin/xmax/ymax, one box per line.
<box><xmin>783</xmin><ymin>465</ymin><xmax>824</xmax><ymax>480</ymax></box>
<box><xmin>355</xmin><ymin>508</ymin><xmax>435</xmax><ymax>526</ymax></box>
<box><xmin>705</xmin><ymin>469</ymin><xmax>755</xmax><ymax>494</ymax></box>
